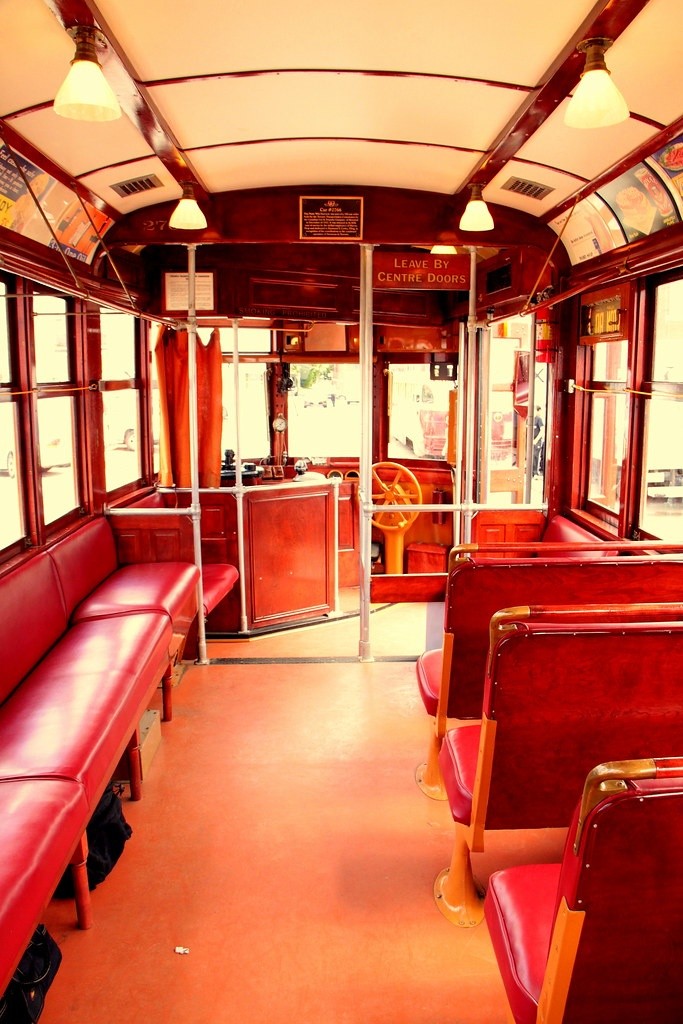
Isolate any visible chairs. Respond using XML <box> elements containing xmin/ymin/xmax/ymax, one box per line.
<box><xmin>109</xmin><ymin>486</ymin><xmax>239</xmax><ymax>618</ymax></box>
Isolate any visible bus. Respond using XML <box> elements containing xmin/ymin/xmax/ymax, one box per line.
<box><xmin>390</xmin><ymin>338</ymin><xmax>514</xmax><ymax>462</ymax></box>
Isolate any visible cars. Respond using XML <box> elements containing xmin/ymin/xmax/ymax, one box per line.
<box><xmin>0</xmin><ymin>418</ymin><xmax>70</xmax><ymax>476</ymax></box>
<box><xmin>103</xmin><ymin>406</ymin><xmax>159</xmax><ymax>451</ymax></box>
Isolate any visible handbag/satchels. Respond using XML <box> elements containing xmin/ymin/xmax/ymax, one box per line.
<box><xmin>54</xmin><ymin>784</ymin><xmax>133</xmax><ymax>899</ymax></box>
<box><xmin>0</xmin><ymin>922</ymin><xmax>61</xmax><ymax>1024</ymax></box>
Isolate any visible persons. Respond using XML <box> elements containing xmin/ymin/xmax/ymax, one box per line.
<box><xmin>512</xmin><ymin>401</ymin><xmax>547</xmax><ymax>479</ymax></box>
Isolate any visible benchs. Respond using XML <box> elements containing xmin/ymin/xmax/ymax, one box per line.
<box><xmin>0</xmin><ymin>516</ymin><xmax>201</xmax><ymax>1000</ymax></box>
<box><xmin>481</xmin><ymin>757</ymin><xmax>682</xmax><ymax>1024</ymax></box>
<box><xmin>416</xmin><ymin>538</ymin><xmax>683</xmax><ymax>800</ymax></box>
<box><xmin>432</xmin><ymin>600</ymin><xmax>683</xmax><ymax>927</ymax></box>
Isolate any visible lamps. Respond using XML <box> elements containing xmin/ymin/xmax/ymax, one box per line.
<box><xmin>169</xmin><ymin>180</ymin><xmax>207</xmax><ymax>230</ymax></box>
<box><xmin>53</xmin><ymin>23</ymin><xmax>122</xmax><ymax>122</ymax></box>
<box><xmin>564</xmin><ymin>34</ymin><xmax>630</xmax><ymax>129</ymax></box>
<box><xmin>459</xmin><ymin>179</ymin><xmax>495</xmax><ymax>232</ymax></box>
